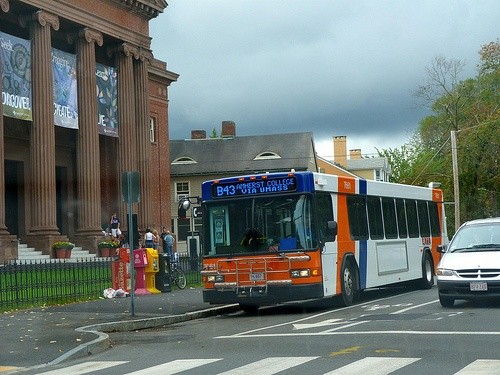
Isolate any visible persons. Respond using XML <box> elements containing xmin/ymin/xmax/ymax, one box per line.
<box><xmin>143</xmin><ymin>227</ymin><xmax>175</xmax><ymax>256</ymax></box>
<box><xmin>110</xmin><ymin>213</ymin><xmax>122</xmax><ymax>239</ymax></box>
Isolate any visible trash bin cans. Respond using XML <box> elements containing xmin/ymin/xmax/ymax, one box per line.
<box><xmin>112</xmin><ymin>247</ymin><xmax>130</xmax><ymax>294</ymax></box>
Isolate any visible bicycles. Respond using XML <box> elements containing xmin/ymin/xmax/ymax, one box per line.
<box><xmin>170</xmin><ymin>260</ymin><xmax>187</xmax><ymax>290</ymax></box>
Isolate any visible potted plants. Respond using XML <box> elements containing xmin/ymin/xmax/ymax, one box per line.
<box><xmin>99</xmin><ymin>241</ymin><xmax>117</xmax><ymax>256</ymax></box>
<box><xmin>52</xmin><ymin>241</ymin><xmax>75</xmax><ymax>258</ymax></box>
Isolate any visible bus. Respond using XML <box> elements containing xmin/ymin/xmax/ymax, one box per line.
<box><xmin>179</xmin><ymin>169</ymin><xmax>448</xmax><ymax>313</ymax></box>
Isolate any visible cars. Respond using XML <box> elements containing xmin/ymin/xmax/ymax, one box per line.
<box><xmin>435</xmin><ymin>218</ymin><xmax>500</xmax><ymax>309</ymax></box>
